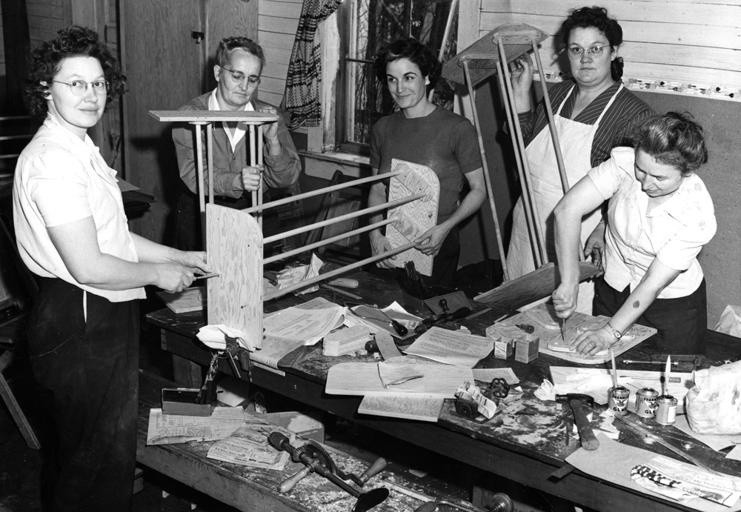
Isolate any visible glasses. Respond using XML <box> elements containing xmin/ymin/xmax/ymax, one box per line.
<box><xmin>49</xmin><ymin>79</ymin><xmax>107</xmax><ymax>93</ymax></box>
<box><xmin>221</xmin><ymin>66</ymin><xmax>259</xmax><ymax>86</ymax></box>
<box><xmin>568</xmin><ymin>45</ymin><xmax>608</xmax><ymax>58</ymax></box>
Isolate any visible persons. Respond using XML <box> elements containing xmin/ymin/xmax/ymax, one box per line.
<box><xmin>551</xmin><ymin>108</ymin><xmax>719</xmax><ymax>362</ymax></box>
<box><xmin>170</xmin><ymin>35</ymin><xmax>303</xmax><ymax>275</ymax></box>
<box><xmin>365</xmin><ymin>39</ymin><xmax>486</xmax><ymax>299</ymax></box>
<box><xmin>498</xmin><ymin>5</ymin><xmax>656</xmax><ymax>315</ymax></box>
<box><xmin>9</xmin><ymin>25</ymin><xmax>216</xmax><ymax>510</ymax></box>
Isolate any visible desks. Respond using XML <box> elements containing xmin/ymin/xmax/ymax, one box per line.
<box><xmin>132</xmin><ymin>244</ymin><xmax>741</xmax><ymax>512</ymax></box>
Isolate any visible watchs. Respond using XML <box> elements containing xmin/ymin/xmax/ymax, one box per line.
<box><xmin>607</xmin><ymin>323</ymin><xmax>623</xmax><ymax>340</ymax></box>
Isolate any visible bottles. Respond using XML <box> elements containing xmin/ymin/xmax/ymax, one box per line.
<box><xmin>608</xmin><ymin>386</ymin><xmax>676</xmax><ymax>427</ymax></box>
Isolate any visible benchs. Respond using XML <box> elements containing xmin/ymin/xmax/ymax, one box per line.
<box><xmin>135</xmin><ymin>365</ymin><xmax>488</xmax><ymax>512</ymax></box>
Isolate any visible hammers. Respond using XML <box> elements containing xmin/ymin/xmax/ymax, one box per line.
<box><xmin>556</xmin><ymin>393</ymin><xmax>600</xmax><ymax>450</ymax></box>
